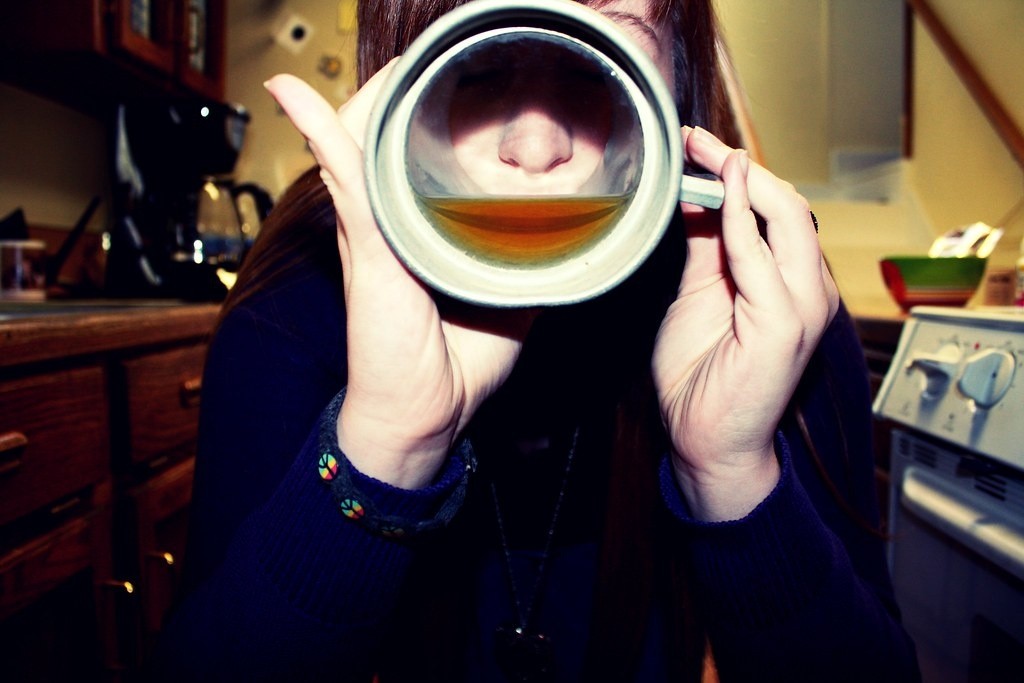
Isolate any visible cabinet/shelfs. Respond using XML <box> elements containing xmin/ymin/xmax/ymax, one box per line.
<box><xmin>0</xmin><ymin>0</ymin><xmax>226</xmax><ymax>119</ymax></box>
<box><xmin>0</xmin><ymin>297</ymin><xmax>219</xmax><ymax>683</ymax></box>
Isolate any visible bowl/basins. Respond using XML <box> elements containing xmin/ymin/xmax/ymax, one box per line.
<box><xmin>878</xmin><ymin>255</ymin><xmax>986</xmax><ymax>313</ymax></box>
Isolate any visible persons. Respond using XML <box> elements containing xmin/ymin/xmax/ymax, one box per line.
<box><xmin>159</xmin><ymin>0</ymin><xmax>926</xmax><ymax>683</ymax></box>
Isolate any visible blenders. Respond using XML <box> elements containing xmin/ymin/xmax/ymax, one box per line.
<box><xmin>99</xmin><ymin>86</ymin><xmax>252</xmax><ymax>302</ymax></box>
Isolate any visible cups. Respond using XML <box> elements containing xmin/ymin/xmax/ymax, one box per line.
<box><xmin>0</xmin><ymin>241</ymin><xmax>48</xmax><ymax>304</ymax></box>
<box><xmin>364</xmin><ymin>0</ymin><xmax>726</xmax><ymax>309</ymax></box>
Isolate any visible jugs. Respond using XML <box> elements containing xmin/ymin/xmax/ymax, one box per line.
<box><xmin>199</xmin><ymin>180</ymin><xmax>273</xmax><ymax>241</ymax></box>
